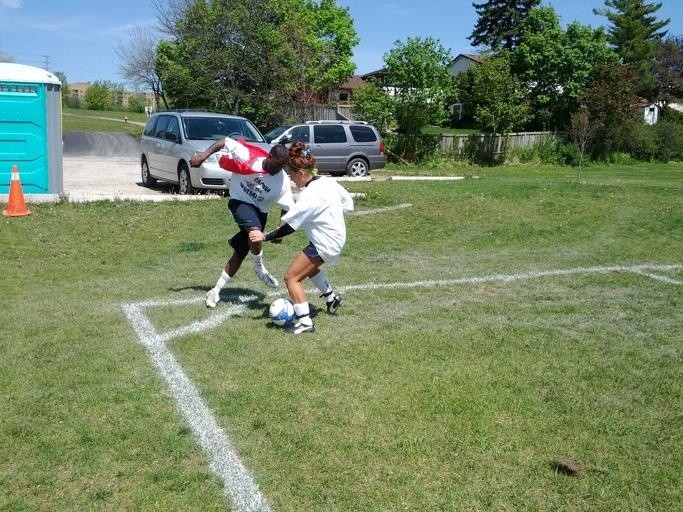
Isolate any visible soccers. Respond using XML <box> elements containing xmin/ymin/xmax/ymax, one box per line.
<box><xmin>269</xmin><ymin>299</ymin><xmax>294</xmax><ymax>327</ymax></box>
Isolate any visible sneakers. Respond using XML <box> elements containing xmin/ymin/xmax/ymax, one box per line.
<box><xmin>325</xmin><ymin>295</ymin><xmax>343</xmax><ymax>314</ymax></box>
<box><xmin>255</xmin><ymin>270</ymin><xmax>278</xmax><ymax>288</ymax></box>
<box><xmin>285</xmin><ymin>322</ymin><xmax>315</xmax><ymax>335</ymax></box>
<box><xmin>206</xmin><ymin>289</ymin><xmax>220</xmax><ymax>308</ymax></box>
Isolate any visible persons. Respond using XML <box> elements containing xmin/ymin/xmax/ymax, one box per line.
<box><xmin>249</xmin><ymin>142</ymin><xmax>355</xmax><ymax>334</ymax></box>
<box><xmin>190</xmin><ymin>137</ymin><xmax>294</xmax><ymax>309</ymax></box>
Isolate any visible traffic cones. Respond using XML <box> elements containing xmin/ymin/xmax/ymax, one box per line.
<box><xmin>2</xmin><ymin>165</ymin><xmax>32</xmax><ymax>218</ymax></box>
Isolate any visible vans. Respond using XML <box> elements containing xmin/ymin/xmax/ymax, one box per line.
<box><xmin>138</xmin><ymin>109</ymin><xmax>272</xmax><ymax>195</ymax></box>
<box><xmin>262</xmin><ymin>119</ymin><xmax>386</xmax><ymax>178</ymax></box>
